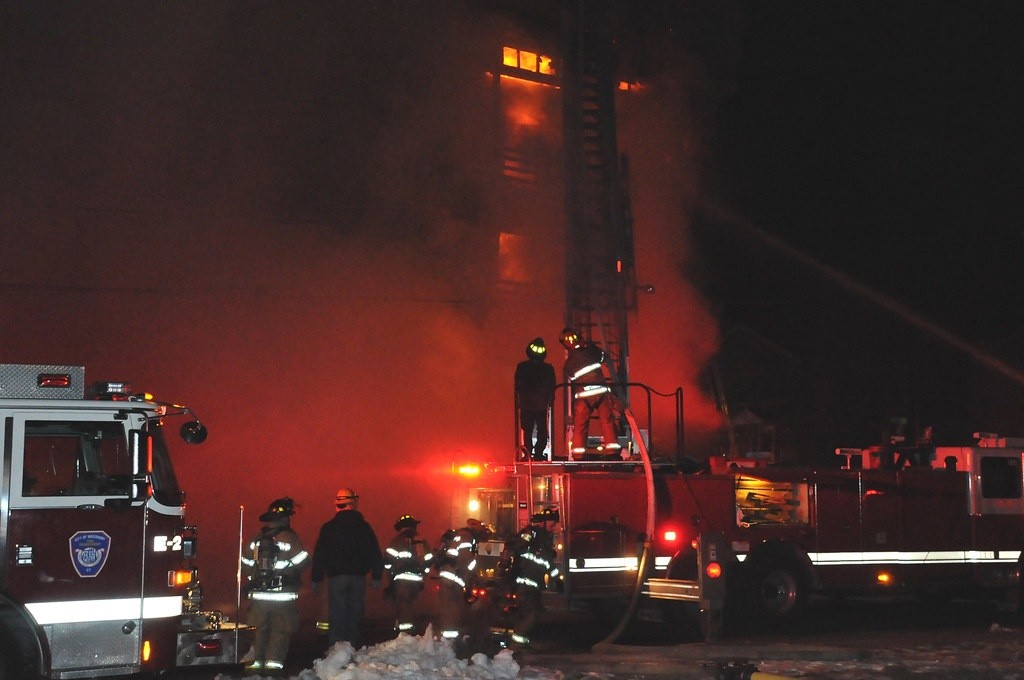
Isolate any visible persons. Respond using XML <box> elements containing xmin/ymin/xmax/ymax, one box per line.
<box><xmin>514</xmin><ymin>337</ymin><xmax>556</xmax><ymax>462</ymax></box>
<box><xmin>384</xmin><ymin>513</ymin><xmax>425</xmax><ymax>637</ymax></box>
<box><xmin>310</xmin><ymin>488</ymin><xmax>384</xmax><ymax>650</ymax></box>
<box><xmin>559</xmin><ymin>326</ymin><xmax>623</xmax><ymax>462</ymax></box>
<box><xmin>500</xmin><ymin>508</ymin><xmax>559</xmax><ymax>652</ymax></box>
<box><xmin>241</xmin><ymin>497</ymin><xmax>311</xmax><ymax>680</ymax></box>
<box><xmin>437</xmin><ymin>518</ymin><xmax>492</xmax><ymax>641</ymax></box>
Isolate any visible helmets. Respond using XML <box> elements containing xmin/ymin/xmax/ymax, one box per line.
<box><xmin>394</xmin><ymin>515</ymin><xmax>421</xmax><ymax>531</ymax></box>
<box><xmin>530</xmin><ymin>510</ymin><xmax>557</xmax><ymax>523</ymax></box>
<box><xmin>335</xmin><ymin>488</ymin><xmax>358</xmax><ymax>505</ymax></box>
<box><xmin>559</xmin><ymin>328</ymin><xmax>580</xmax><ymax>349</ymax></box>
<box><xmin>467</xmin><ymin>518</ymin><xmax>491</xmax><ymax>534</ymax></box>
<box><xmin>527</xmin><ymin>337</ymin><xmax>546</xmax><ymax>358</ymax></box>
<box><xmin>259</xmin><ymin>501</ymin><xmax>297</xmax><ymax>521</ymax></box>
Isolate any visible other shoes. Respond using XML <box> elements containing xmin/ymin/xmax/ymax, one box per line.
<box><xmin>534</xmin><ymin>454</ymin><xmax>547</xmax><ymax>461</ymax></box>
<box><xmin>511</xmin><ymin>639</ymin><xmax>541</xmax><ymax>648</ymax></box>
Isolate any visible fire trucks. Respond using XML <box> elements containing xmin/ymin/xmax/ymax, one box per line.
<box><xmin>0</xmin><ymin>362</ymin><xmax>257</xmax><ymax>680</ymax></box>
<box><xmin>469</xmin><ymin>1</ymin><xmax>1024</xmax><ymax>634</ymax></box>
